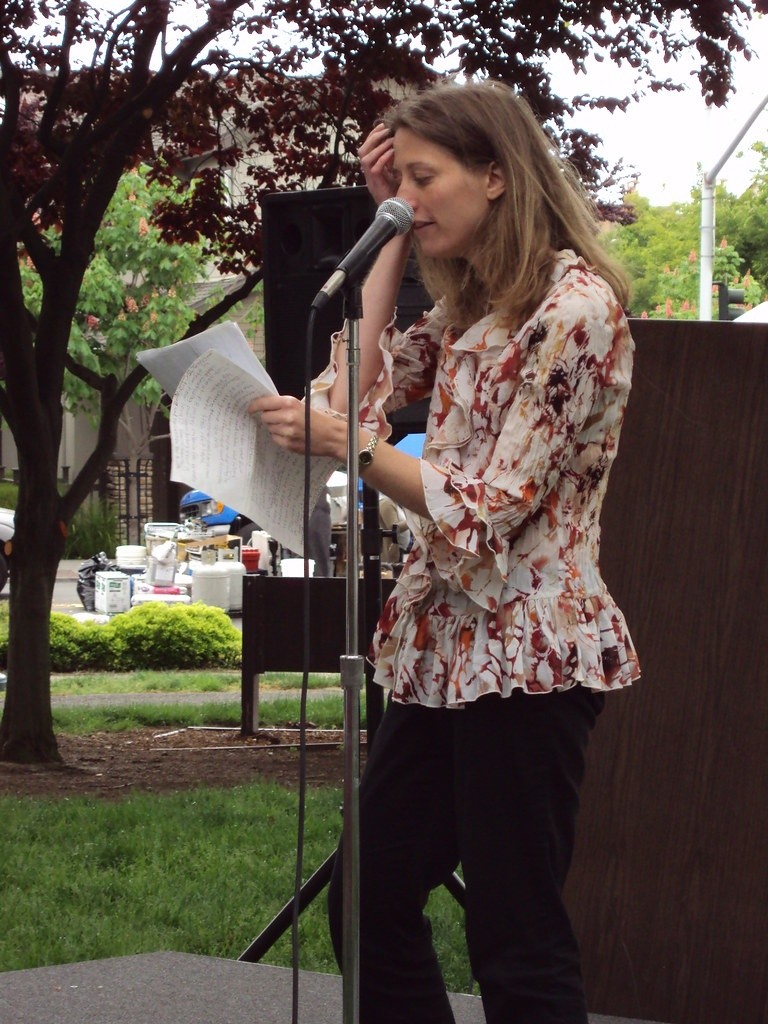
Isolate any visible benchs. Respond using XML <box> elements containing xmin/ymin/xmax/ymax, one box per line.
<box><xmin>241</xmin><ymin>573</ymin><xmax>397</xmax><ymax>733</ymax></box>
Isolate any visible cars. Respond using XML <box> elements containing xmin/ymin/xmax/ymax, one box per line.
<box><xmin>179</xmin><ymin>434</ymin><xmax>426</xmax><ymax>544</ymax></box>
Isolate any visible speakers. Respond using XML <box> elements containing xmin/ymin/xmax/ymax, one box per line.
<box><xmin>260</xmin><ymin>184</ymin><xmax>432</xmax><ymax>450</ymax></box>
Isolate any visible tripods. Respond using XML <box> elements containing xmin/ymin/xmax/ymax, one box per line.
<box><xmin>238</xmin><ymin>486</ymin><xmax>468</xmax><ymax>963</ymax></box>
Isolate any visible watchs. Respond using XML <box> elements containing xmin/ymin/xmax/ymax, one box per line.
<box><xmin>358</xmin><ymin>434</ymin><xmax>380</xmax><ymax>474</ymax></box>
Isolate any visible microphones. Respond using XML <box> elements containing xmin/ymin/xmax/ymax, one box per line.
<box><xmin>311</xmin><ymin>197</ymin><xmax>416</xmax><ymax>314</ymax></box>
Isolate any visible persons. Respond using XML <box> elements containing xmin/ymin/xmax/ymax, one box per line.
<box><xmin>248</xmin><ymin>83</ymin><xmax>642</xmax><ymax>1024</ymax></box>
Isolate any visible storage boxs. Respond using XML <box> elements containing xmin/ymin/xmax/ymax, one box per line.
<box><xmin>94</xmin><ymin>533</ymin><xmax>260</xmax><ymax>621</ymax></box>
